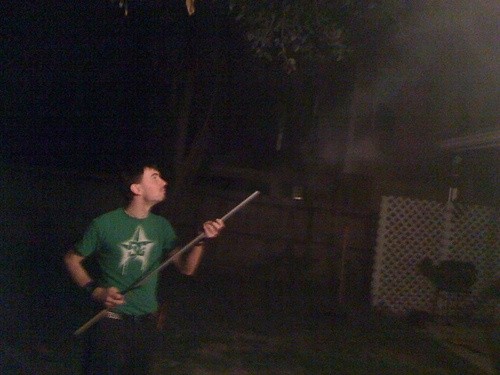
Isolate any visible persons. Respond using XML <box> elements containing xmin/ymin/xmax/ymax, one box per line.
<box><xmin>62</xmin><ymin>161</ymin><xmax>226</xmax><ymax>375</ymax></box>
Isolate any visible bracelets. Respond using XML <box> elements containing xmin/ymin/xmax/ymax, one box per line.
<box><xmin>81</xmin><ymin>280</ymin><xmax>97</xmax><ymax>297</ymax></box>
<box><xmin>195</xmin><ymin>239</ymin><xmax>208</xmax><ymax>248</ymax></box>
